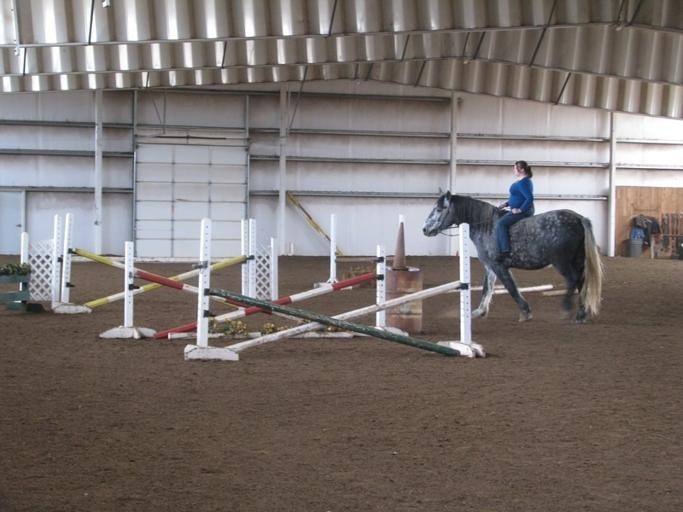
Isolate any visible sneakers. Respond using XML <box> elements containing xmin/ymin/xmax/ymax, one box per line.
<box><xmin>496</xmin><ymin>252</ymin><xmax>512</xmax><ymax>262</ymax></box>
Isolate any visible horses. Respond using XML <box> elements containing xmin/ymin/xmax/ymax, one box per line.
<box><xmin>423</xmin><ymin>187</ymin><xmax>605</xmax><ymax>324</ymax></box>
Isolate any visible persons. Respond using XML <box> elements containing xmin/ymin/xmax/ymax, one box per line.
<box><xmin>495</xmin><ymin>160</ymin><xmax>535</xmax><ymax>263</ymax></box>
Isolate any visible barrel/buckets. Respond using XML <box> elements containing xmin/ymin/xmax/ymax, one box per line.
<box><xmin>629</xmin><ymin>239</ymin><xmax>642</xmax><ymax>258</ymax></box>
<box><xmin>385</xmin><ymin>265</ymin><xmax>426</xmax><ymax>335</ymax></box>
